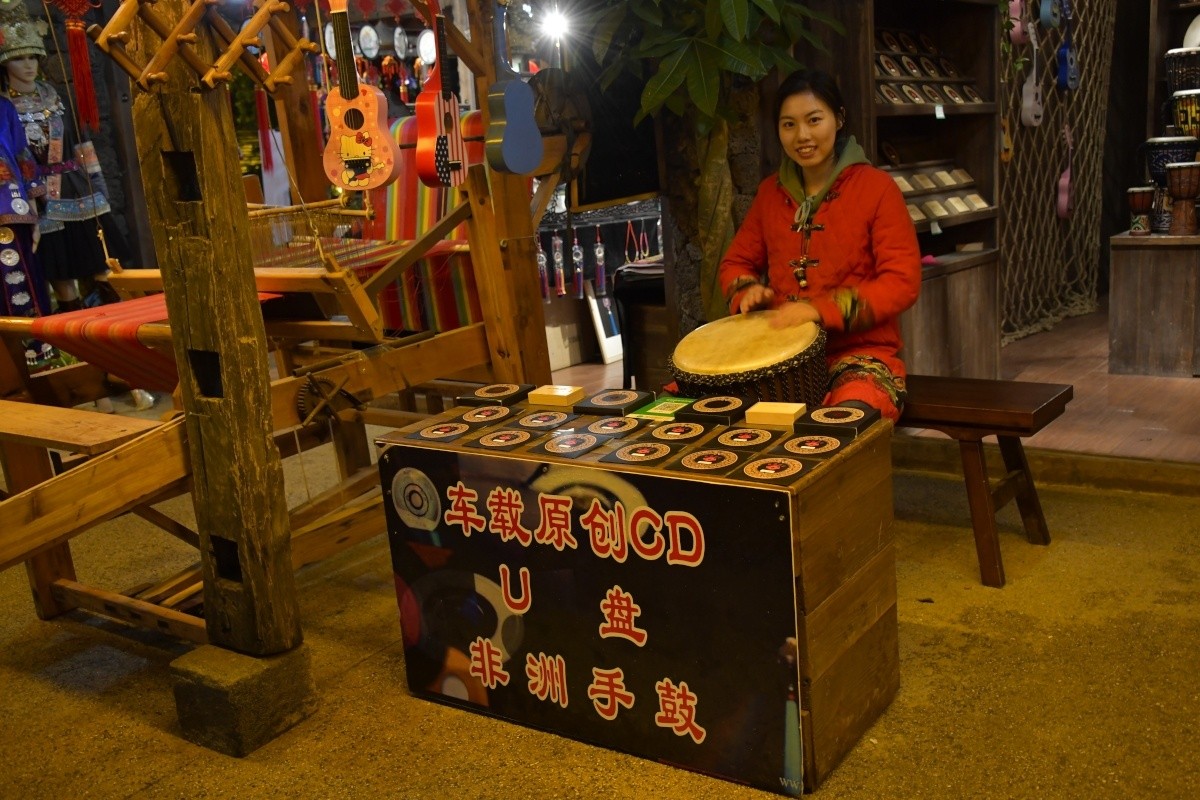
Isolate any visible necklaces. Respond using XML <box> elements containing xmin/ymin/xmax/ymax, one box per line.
<box><xmin>793</xmin><ymin>167</ymin><xmax>806</xmax><ymax>288</ymax></box>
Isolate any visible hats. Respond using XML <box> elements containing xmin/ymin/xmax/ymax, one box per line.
<box><xmin>0</xmin><ymin>1</ymin><xmax>49</xmax><ymax>62</ymax></box>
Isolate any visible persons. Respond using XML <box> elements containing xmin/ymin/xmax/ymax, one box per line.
<box><xmin>662</xmin><ymin>68</ymin><xmax>922</xmax><ymax>425</ymax></box>
<box><xmin>0</xmin><ymin>94</ymin><xmax>61</xmax><ymax>374</ymax></box>
<box><xmin>0</xmin><ymin>0</ymin><xmax>129</xmax><ymax>312</ymax></box>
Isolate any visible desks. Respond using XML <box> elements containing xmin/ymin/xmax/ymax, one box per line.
<box><xmin>375</xmin><ymin>384</ymin><xmax>900</xmax><ymax>794</ymax></box>
<box><xmin>1107</xmin><ymin>230</ymin><xmax>1200</xmax><ymax>378</ymax></box>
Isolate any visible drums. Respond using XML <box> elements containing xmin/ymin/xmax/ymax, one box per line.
<box><xmin>1164</xmin><ymin>46</ymin><xmax>1200</xmax><ymax>91</ymax></box>
<box><xmin>667</xmin><ymin>307</ymin><xmax>828</xmax><ymax>414</ymax></box>
<box><xmin>1126</xmin><ymin>186</ymin><xmax>1156</xmax><ymax>235</ymax></box>
<box><xmin>1145</xmin><ymin>135</ymin><xmax>1198</xmax><ymax>233</ymax></box>
<box><xmin>1170</xmin><ymin>88</ymin><xmax>1199</xmax><ymax>137</ymax></box>
<box><xmin>1166</xmin><ymin>160</ymin><xmax>1200</xmax><ymax>236</ymax></box>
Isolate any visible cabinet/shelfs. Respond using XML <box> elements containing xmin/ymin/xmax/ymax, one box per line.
<box><xmin>793</xmin><ymin>0</ymin><xmax>1001</xmax><ymax>378</ymax></box>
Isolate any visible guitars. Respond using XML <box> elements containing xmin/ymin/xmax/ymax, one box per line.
<box><xmin>1039</xmin><ymin>0</ymin><xmax>1062</xmax><ymax>28</ymax></box>
<box><xmin>320</xmin><ymin>1</ymin><xmax>403</xmax><ymax>192</ymax></box>
<box><xmin>1001</xmin><ymin>76</ymin><xmax>1016</xmax><ymax>163</ymax></box>
<box><xmin>1056</xmin><ymin>124</ymin><xmax>1076</xmax><ymax>220</ymax></box>
<box><xmin>1057</xmin><ymin>0</ymin><xmax>1079</xmax><ymax>90</ymax></box>
<box><xmin>1006</xmin><ymin>0</ymin><xmax>1030</xmax><ymax>45</ymax></box>
<box><xmin>412</xmin><ymin>0</ymin><xmax>470</xmax><ymax>189</ymax></box>
<box><xmin>1021</xmin><ymin>21</ymin><xmax>1044</xmax><ymax>128</ymax></box>
<box><xmin>485</xmin><ymin>0</ymin><xmax>546</xmax><ymax>176</ymax></box>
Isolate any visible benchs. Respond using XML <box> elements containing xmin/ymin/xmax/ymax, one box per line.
<box><xmin>895</xmin><ymin>375</ymin><xmax>1074</xmax><ymax>587</ymax></box>
